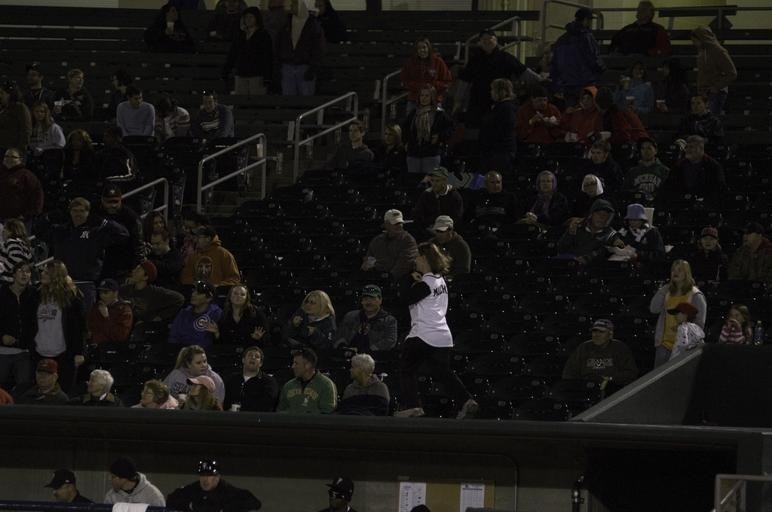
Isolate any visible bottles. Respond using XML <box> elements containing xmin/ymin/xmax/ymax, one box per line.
<box><xmin>753</xmin><ymin>320</ymin><xmax>764</xmax><ymax>346</ymax></box>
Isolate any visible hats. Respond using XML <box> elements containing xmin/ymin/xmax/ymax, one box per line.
<box><xmin>103</xmin><ymin>184</ymin><xmax>121</xmax><ymax>201</ymax></box>
<box><xmin>93</xmin><ymin>279</ymin><xmax>118</xmax><ymax>291</ymax></box>
<box><xmin>110</xmin><ymin>458</ymin><xmax>137</xmax><ymax>478</ymax></box>
<box><xmin>700</xmin><ymin>222</ymin><xmax>762</xmax><ymax>240</ymax></box>
<box><xmin>186</xmin><ymin>375</ymin><xmax>216</xmax><ymax>393</ymax></box>
<box><xmin>143</xmin><ymin>260</ymin><xmax>157</xmax><ymax>281</ymax></box>
<box><xmin>44</xmin><ymin>469</ymin><xmax>75</xmax><ymax>488</ymax></box>
<box><xmin>192</xmin><ymin>457</ymin><xmax>219</xmax><ymax>475</ymax></box>
<box><xmin>384</xmin><ymin>167</ymin><xmax>454</xmax><ymax>232</ymax></box>
<box><xmin>192</xmin><ymin>225</ymin><xmax>214</xmax><ymax>235</ymax></box>
<box><xmin>362</xmin><ymin>285</ymin><xmax>382</xmax><ymax>298</ymax></box>
<box><xmin>591</xmin><ymin>199</ymin><xmax>648</xmax><ymax>220</ymax></box>
<box><xmin>325</xmin><ymin>478</ymin><xmax>353</xmax><ymax>493</ymax></box>
<box><xmin>592</xmin><ymin>319</ymin><xmax>614</xmax><ymax>335</ymax></box>
<box><xmin>667</xmin><ymin>303</ymin><xmax>698</xmax><ymax>318</ymax></box>
<box><xmin>37</xmin><ymin>359</ymin><xmax>58</xmax><ymax>372</ymax></box>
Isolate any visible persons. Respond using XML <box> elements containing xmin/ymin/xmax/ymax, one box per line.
<box><xmin>1</xmin><ymin>62</ymin><xmax>772</xmax><ymax>416</ymax></box>
<box><xmin>323</xmin><ymin>476</ymin><xmax>355</xmax><ymax>511</ymax></box>
<box><xmin>167</xmin><ymin>457</ymin><xmax>262</xmax><ymax>511</ymax></box>
<box><xmin>455</xmin><ymin>1</ymin><xmax>738</xmax><ymax>115</ymax></box>
<box><xmin>43</xmin><ymin>468</ymin><xmax>96</xmax><ymax>504</ymax></box>
<box><xmin>412</xmin><ymin>504</ymin><xmax>431</xmax><ymax>511</ymax></box>
<box><xmin>405</xmin><ymin>36</ymin><xmax>452</xmax><ymax>121</ymax></box>
<box><xmin>104</xmin><ymin>458</ymin><xmax>165</xmax><ymax>506</ymax></box>
<box><xmin>143</xmin><ymin>1</ymin><xmax>345</xmax><ymax>96</ymax></box>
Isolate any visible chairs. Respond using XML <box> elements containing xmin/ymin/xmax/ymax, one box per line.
<box><xmin>0</xmin><ymin>0</ymin><xmax>772</xmax><ymax>428</ymax></box>
